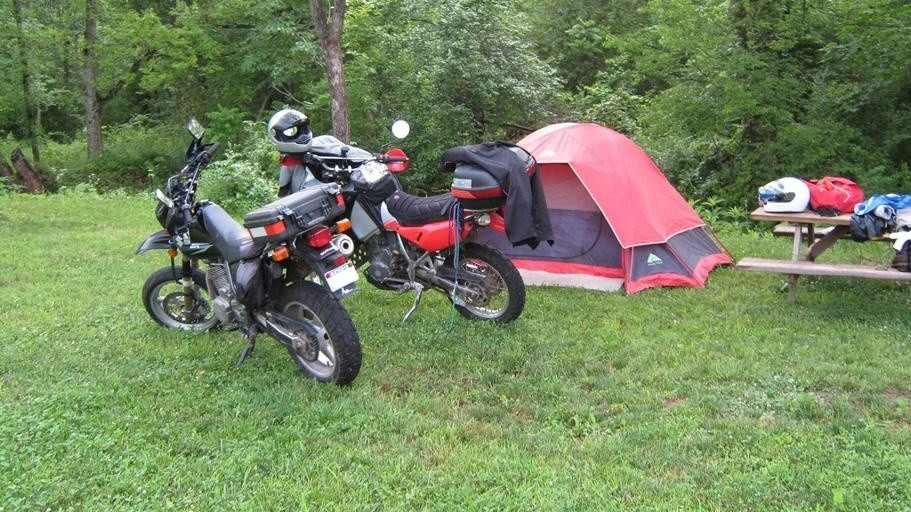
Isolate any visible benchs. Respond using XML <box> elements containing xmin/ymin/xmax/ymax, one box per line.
<box><xmin>732</xmin><ymin>225</ymin><xmax>911</xmax><ymax>284</ymax></box>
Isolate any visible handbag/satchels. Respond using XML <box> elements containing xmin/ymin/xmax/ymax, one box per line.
<box><xmin>801</xmin><ymin>176</ymin><xmax>864</xmax><ymax>214</ymax></box>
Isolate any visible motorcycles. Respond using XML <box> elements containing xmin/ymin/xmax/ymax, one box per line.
<box><xmin>132</xmin><ymin>116</ymin><xmax>367</xmax><ymax>389</ymax></box>
<box><xmin>267</xmin><ymin>103</ymin><xmax>542</xmax><ymax>329</ymax></box>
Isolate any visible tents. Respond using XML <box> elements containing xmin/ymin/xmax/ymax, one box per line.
<box><xmin>470</xmin><ymin>121</ymin><xmax>738</xmax><ymax>295</ymax></box>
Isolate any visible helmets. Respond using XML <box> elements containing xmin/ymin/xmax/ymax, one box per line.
<box><xmin>266</xmin><ymin>109</ymin><xmax>314</xmax><ymax>155</ymax></box>
<box><xmin>757</xmin><ymin>176</ymin><xmax>811</xmax><ymax>214</ymax></box>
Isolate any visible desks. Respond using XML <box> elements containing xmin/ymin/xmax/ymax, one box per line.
<box><xmin>749</xmin><ymin>206</ymin><xmax>851</xmax><ymax>302</ymax></box>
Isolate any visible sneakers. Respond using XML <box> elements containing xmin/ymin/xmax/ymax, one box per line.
<box><xmin>890</xmin><ymin>251</ymin><xmax>909</xmax><ymax>272</ymax></box>
